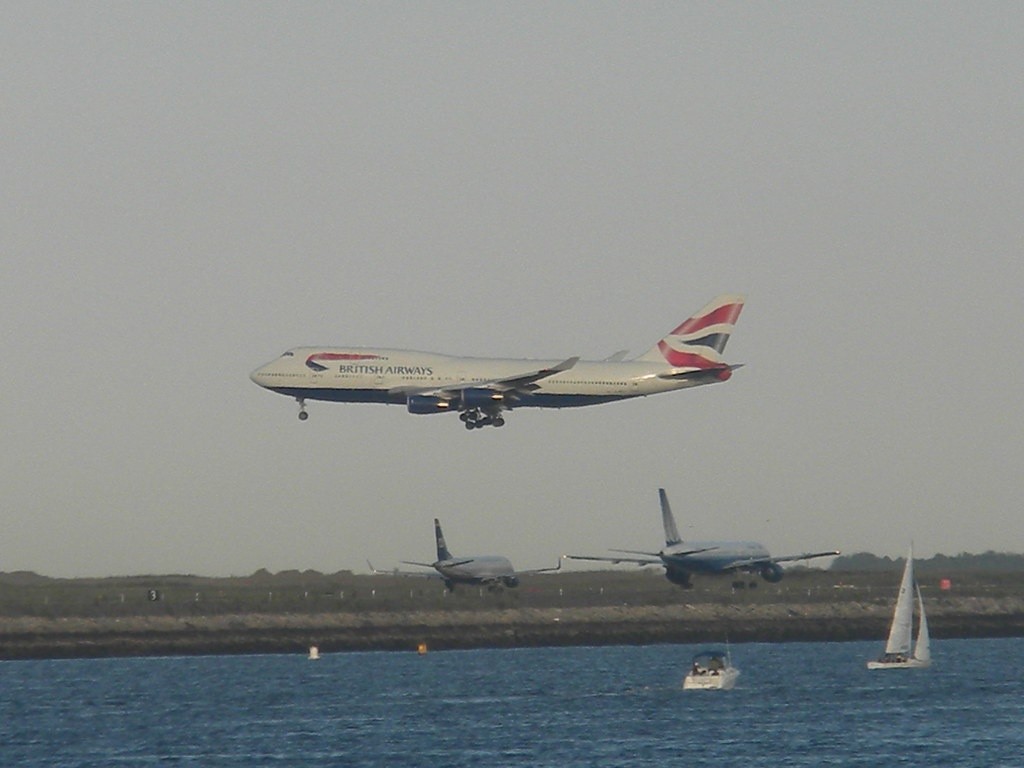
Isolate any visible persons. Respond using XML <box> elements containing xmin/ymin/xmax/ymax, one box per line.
<box><xmin>693</xmin><ymin>656</ymin><xmax>723</xmax><ymax>675</ymax></box>
<box><xmin>881</xmin><ymin>653</ymin><xmax>908</xmax><ymax>662</ymax></box>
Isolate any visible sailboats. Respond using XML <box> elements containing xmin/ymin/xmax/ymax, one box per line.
<box><xmin>866</xmin><ymin>540</ymin><xmax>933</xmax><ymax>669</ymax></box>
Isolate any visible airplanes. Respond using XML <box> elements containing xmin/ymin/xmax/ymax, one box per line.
<box><xmin>248</xmin><ymin>265</ymin><xmax>767</xmax><ymax>432</ymax></box>
<box><xmin>402</xmin><ymin>517</ymin><xmax>562</xmax><ymax>596</ymax></box>
<box><xmin>566</xmin><ymin>487</ymin><xmax>841</xmax><ymax>590</ymax></box>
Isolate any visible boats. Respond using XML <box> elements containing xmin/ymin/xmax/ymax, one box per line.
<box><xmin>683</xmin><ymin>650</ymin><xmax>741</xmax><ymax>690</ymax></box>
